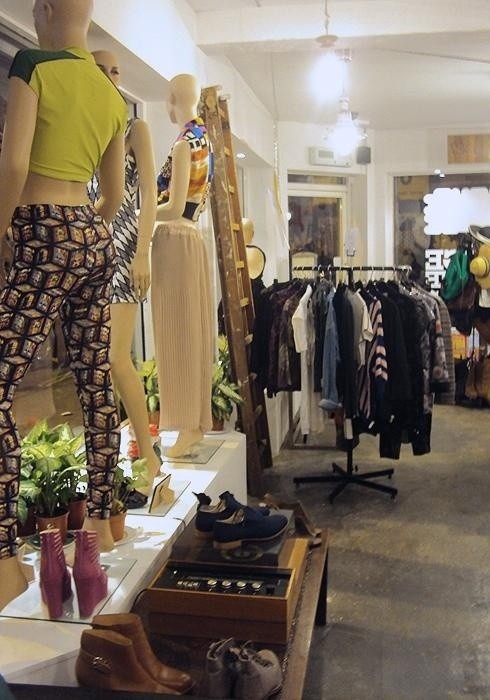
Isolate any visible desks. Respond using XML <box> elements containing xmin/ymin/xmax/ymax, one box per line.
<box><xmin>134</xmin><ymin>516</ymin><xmax>329</xmax><ymax>699</ymax></box>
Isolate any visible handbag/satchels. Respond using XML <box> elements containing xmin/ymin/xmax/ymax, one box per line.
<box><xmin>438</xmin><ymin>232</ymin><xmax>481</xmax><ymax>337</ymax></box>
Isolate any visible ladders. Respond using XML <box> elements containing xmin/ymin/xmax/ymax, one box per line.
<box><xmin>199</xmin><ymin>86</ymin><xmax>272</xmax><ymax>498</ymax></box>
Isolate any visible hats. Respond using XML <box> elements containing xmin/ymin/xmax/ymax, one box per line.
<box><xmin>469</xmin><ymin>243</ymin><xmax>490</xmax><ymax>290</ymax></box>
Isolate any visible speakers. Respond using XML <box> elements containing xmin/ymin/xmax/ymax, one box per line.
<box><xmin>357</xmin><ymin>147</ymin><xmax>371</xmax><ymax>163</ymax></box>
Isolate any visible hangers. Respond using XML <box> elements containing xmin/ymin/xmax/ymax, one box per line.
<box><xmin>267</xmin><ymin>265</ymin><xmax>428</xmax><ymax>302</ymax></box>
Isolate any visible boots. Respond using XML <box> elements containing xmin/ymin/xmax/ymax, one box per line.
<box><xmin>70</xmin><ymin>527</ymin><xmax>110</xmax><ymax>622</ymax></box>
<box><xmin>229</xmin><ymin>640</ymin><xmax>284</xmax><ymax>700</ymax></box>
<box><xmin>37</xmin><ymin>529</ymin><xmax>73</xmax><ymax>621</ymax></box>
<box><xmin>88</xmin><ymin>612</ymin><xmax>196</xmax><ymax>694</ymax></box>
<box><xmin>72</xmin><ymin>628</ymin><xmax>180</xmax><ymax>696</ymax></box>
<box><xmin>203</xmin><ymin>635</ymin><xmax>236</xmax><ymax>700</ymax></box>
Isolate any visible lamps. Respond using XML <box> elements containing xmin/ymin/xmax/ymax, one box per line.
<box><xmin>305</xmin><ymin>3</ymin><xmax>348</xmax><ymax>111</ymax></box>
<box><xmin>322</xmin><ymin>46</ymin><xmax>366</xmax><ymax>155</ymax></box>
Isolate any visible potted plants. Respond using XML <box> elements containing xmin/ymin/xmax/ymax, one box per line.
<box><xmin>206</xmin><ymin>361</ymin><xmax>246</xmax><ymax>432</ymax></box>
<box><xmin>16</xmin><ymin>410</ymin><xmax>148</xmax><ymax>542</ymax></box>
<box><xmin>133</xmin><ymin>357</ymin><xmax>160</xmax><ymax>429</ymax></box>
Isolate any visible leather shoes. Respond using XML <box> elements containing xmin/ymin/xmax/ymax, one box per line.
<box><xmin>190</xmin><ymin>489</ymin><xmax>271</xmax><ymax>540</ymax></box>
<box><xmin>210</xmin><ymin>507</ymin><xmax>289</xmax><ymax>551</ymax></box>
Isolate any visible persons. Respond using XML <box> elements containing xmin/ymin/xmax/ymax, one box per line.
<box><xmin>88</xmin><ymin>50</ymin><xmax>160</xmax><ymax>496</ymax></box>
<box><xmin>129</xmin><ymin>74</ymin><xmax>214</xmax><ymax>458</ymax></box>
<box><xmin>218</xmin><ymin>217</ymin><xmax>266</xmax><ymax>337</ymax></box>
<box><xmin>1</xmin><ymin>0</ymin><xmax>129</xmax><ymax>614</ymax></box>
